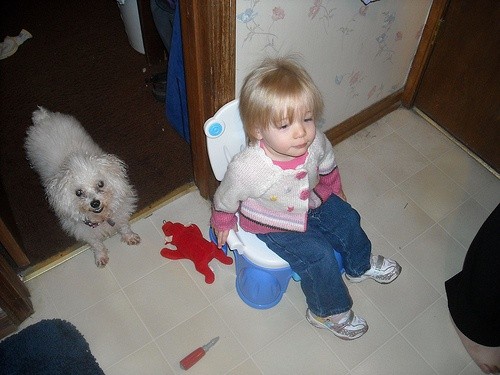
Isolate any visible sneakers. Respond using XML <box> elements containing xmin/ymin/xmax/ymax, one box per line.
<box><xmin>306</xmin><ymin>308</ymin><xmax>368</xmax><ymax>340</ymax></box>
<box><xmin>345</xmin><ymin>254</ymin><xmax>402</xmax><ymax>284</ymax></box>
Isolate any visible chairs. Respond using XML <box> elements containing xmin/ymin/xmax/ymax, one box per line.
<box><xmin>203</xmin><ymin>98</ymin><xmax>345</xmax><ymax>309</ymax></box>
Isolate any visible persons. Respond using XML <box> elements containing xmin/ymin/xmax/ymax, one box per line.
<box><xmin>210</xmin><ymin>55</ymin><xmax>403</xmax><ymax>341</ymax></box>
<box><xmin>444</xmin><ymin>202</ymin><xmax>500</xmax><ymax>375</ymax></box>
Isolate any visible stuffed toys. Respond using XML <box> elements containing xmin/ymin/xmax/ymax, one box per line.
<box><xmin>160</xmin><ymin>220</ymin><xmax>233</xmax><ymax>283</ymax></box>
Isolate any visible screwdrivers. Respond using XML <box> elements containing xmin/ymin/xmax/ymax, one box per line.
<box><xmin>179</xmin><ymin>337</ymin><xmax>221</xmax><ymax>370</ymax></box>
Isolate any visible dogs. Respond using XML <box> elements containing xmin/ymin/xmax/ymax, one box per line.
<box><xmin>25</xmin><ymin>106</ymin><xmax>141</xmax><ymax>267</ymax></box>
